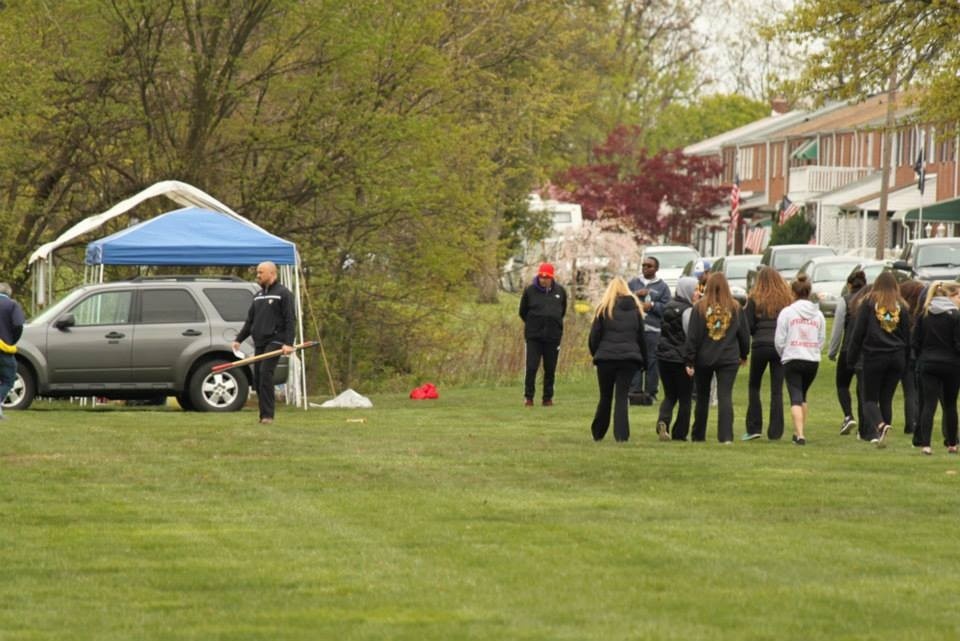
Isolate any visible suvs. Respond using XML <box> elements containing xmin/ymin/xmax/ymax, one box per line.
<box><xmin>0</xmin><ymin>271</ymin><xmax>294</xmax><ymax>415</ymax></box>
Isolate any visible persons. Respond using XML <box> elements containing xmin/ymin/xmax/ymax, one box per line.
<box><xmin>829</xmin><ymin>271</ymin><xmax>867</xmax><ymax>439</ymax></box>
<box><xmin>589</xmin><ymin>275</ymin><xmax>648</xmax><ymax>442</ymax></box>
<box><xmin>683</xmin><ymin>272</ymin><xmax>750</xmax><ymax>444</ymax></box>
<box><xmin>232</xmin><ymin>261</ymin><xmax>297</xmax><ymax>424</ymax></box>
<box><xmin>0</xmin><ymin>282</ymin><xmax>23</xmax><ymax>418</ymax></box>
<box><xmin>628</xmin><ymin>257</ymin><xmax>671</xmax><ymax>406</ymax></box>
<box><xmin>519</xmin><ymin>262</ymin><xmax>567</xmax><ymax>407</ymax></box>
<box><xmin>774</xmin><ymin>274</ymin><xmax>826</xmax><ymax>445</ymax></box>
<box><xmin>744</xmin><ymin>267</ymin><xmax>794</xmax><ymax>439</ymax></box>
<box><xmin>846</xmin><ymin>271</ymin><xmax>960</xmax><ymax>454</ymax></box>
<box><xmin>654</xmin><ymin>276</ymin><xmax>700</xmax><ymax>440</ymax></box>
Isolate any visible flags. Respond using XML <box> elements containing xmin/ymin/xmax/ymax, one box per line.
<box><xmin>779</xmin><ymin>196</ymin><xmax>798</xmax><ymax>225</ymax></box>
<box><xmin>728</xmin><ymin>176</ymin><xmax>740</xmax><ymax>245</ymax></box>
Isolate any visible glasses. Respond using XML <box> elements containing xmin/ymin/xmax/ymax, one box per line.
<box><xmin>642</xmin><ymin>263</ymin><xmax>656</xmax><ymax>269</ymax></box>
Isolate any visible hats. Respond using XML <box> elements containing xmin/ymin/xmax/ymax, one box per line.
<box><xmin>693</xmin><ymin>261</ymin><xmax>711</xmax><ymax>276</ymax></box>
<box><xmin>893</xmin><ymin>261</ymin><xmax>917</xmax><ymax>274</ymax></box>
<box><xmin>538</xmin><ymin>263</ymin><xmax>554</xmax><ymax>278</ymax></box>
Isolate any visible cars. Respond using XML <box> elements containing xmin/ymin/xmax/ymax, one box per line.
<box><xmin>512</xmin><ymin>200</ymin><xmax>960</xmax><ymax>317</ymax></box>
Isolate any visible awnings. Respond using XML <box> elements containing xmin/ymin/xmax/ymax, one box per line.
<box><xmin>792</xmin><ymin>138</ymin><xmax>817</xmax><ymax>159</ymax></box>
<box><xmin>891</xmin><ymin>197</ymin><xmax>960</xmax><ymax>223</ymax></box>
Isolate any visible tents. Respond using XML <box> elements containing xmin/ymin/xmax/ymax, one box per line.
<box><xmin>28</xmin><ymin>180</ymin><xmax>267</xmax><ymax>318</ymax></box>
<box><xmin>78</xmin><ymin>204</ymin><xmax>308</xmax><ymax>409</ymax></box>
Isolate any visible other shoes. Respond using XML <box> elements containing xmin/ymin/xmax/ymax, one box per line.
<box><xmin>0</xmin><ymin>408</ymin><xmax>4</xmax><ymax>419</ymax></box>
<box><xmin>742</xmin><ymin>433</ymin><xmax>760</xmax><ymax>441</ymax></box>
<box><xmin>948</xmin><ymin>446</ymin><xmax>957</xmax><ymax>452</ymax></box>
<box><xmin>524</xmin><ymin>399</ymin><xmax>533</xmax><ymax>406</ymax></box>
<box><xmin>658</xmin><ymin>422</ymin><xmax>670</xmax><ymax>441</ymax></box>
<box><xmin>870</xmin><ymin>439</ymin><xmax>878</xmax><ymax>443</ymax></box>
<box><xmin>840</xmin><ymin>416</ymin><xmax>856</xmax><ymax>435</ymax></box>
<box><xmin>792</xmin><ymin>435</ymin><xmax>805</xmax><ymax>446</ymax></box>
<box><xmin>922</xmin><ymin>447</ymin><xmax>931</xmax><ymax>454</ymax></box>
<box><xmin>879</xmin><ymin>423</ymin><xmax>892</xmax><ymax>446</ymax></box>
<box><xmin>259</xmin><ymin>417</ymin><xmax>272</xmax><ymax>424</ymax></box>
<box><xmin>542</xmin><ymin>399</ymin><xmax>552</xmax><ymax>406</ymax></box>
